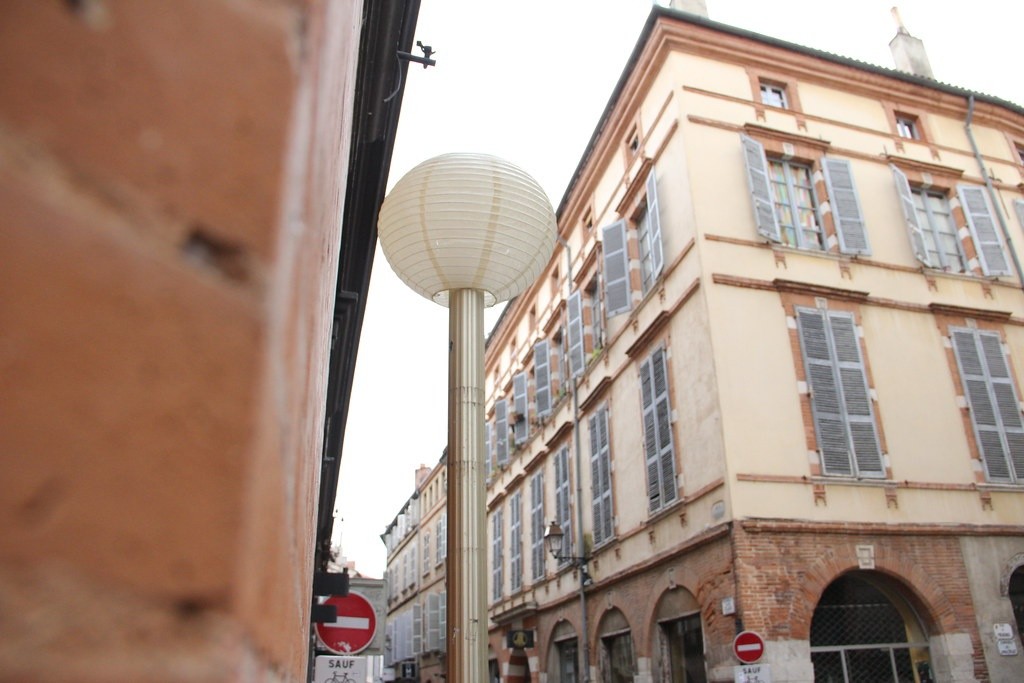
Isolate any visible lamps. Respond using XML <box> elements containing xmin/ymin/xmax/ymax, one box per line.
<box><xmin>544</xmin><ymin>521</ymin><xmax>593</xmax><ymax>587</ymax></box>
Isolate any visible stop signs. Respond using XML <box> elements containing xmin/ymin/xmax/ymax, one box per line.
<box><xmin>733</xmin><ymin>630</ymin><xmax>769</xmax><ymax>665</ymax></box>
<box><xmin>314</xmin><ymin>592</ymin><xmax>379</xmax><ymax>656</ymax></box>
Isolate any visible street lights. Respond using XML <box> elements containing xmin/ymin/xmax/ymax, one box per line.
<box><xmin>538</xmin><ymin>520</ymin><xmax>593</xmax><ymax>682</ymax></box>
<box><xmin>380</xmin><ymin>150</ymin><xmax>562</xmax><ymax>680</ymax></box>
